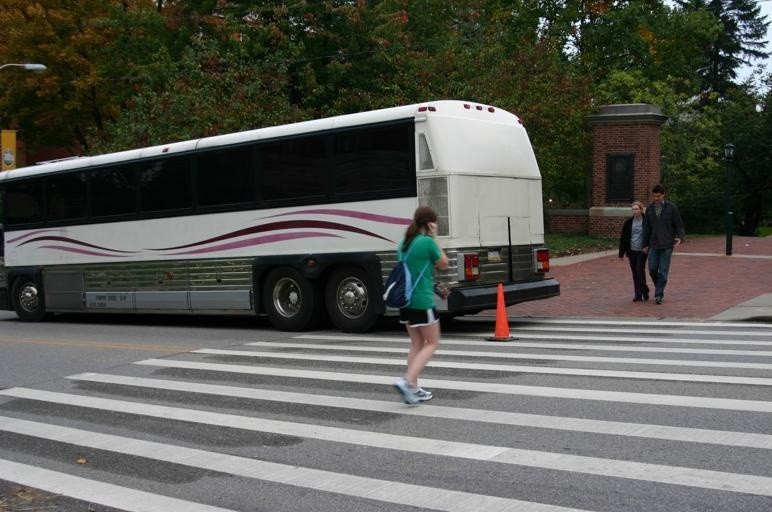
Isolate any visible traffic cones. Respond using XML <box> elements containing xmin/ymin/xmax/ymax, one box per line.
<box><xmin>484</xmin><ymin>284</ymin><xmax>518</xmax><ymax>344</ymax></box>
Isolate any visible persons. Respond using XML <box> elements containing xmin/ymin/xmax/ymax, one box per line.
<box><xmin>641</xmin><ymin>185</ymin><xmax>686</xmax><ymax>304</ymax></box>
<box><xmin>393</xmin><ymin>207</ymin><xmax>448</xmax><ymax>405</ymax></box>
<box><xmin>618</xmin><ymin>201</ymin><xmax>650</xmax><ymax>302</ymax></box>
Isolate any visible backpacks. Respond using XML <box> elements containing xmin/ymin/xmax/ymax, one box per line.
<box><xmin>382</xmin><ymin>235</ymin><xmax>431</xmax><ymax>309</ymax></box>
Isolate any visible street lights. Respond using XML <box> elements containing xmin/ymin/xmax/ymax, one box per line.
<box><xmin>1</xmin><ymin>58</ymin><xmax>48</xmax><ymax>74</ymax></box>
<box><xmin>721</xmin><ymin>143</ymin><xmax>737</xmax><ymax>256</ymax></box>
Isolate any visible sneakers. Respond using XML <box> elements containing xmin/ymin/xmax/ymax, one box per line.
<box><xmin>655</xmin><ymin>295</ymin><xmax>664</xmax><ymax>303</ymax></box>
<box><xmin>394</xmin><ymin>377</ymin><xmax>415</xmax><ymax>404</ymax></box>
<box><xmin>405</xmin><ymin>388</ymin><xmax>432</xmax><ymax>404</ymax></box>
<box><xmin>643</xmin><ymin>287</ymin><xmax>649</xmax><ymax>299</ymax></box>
<box><xmin>633</xmin><ymin>296</ymin><xmax>642</xmax><ymax>301</ymax></box>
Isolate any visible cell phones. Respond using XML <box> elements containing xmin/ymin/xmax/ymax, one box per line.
<box><xmin>425</xmin><ymin>225</ymin><xmax>432</xmax><ymax>231</ymax></box>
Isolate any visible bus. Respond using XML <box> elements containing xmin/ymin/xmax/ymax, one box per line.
<box><xmin>0</xmin><ymin>100</ymin><xmax>561</xmax><ymax>336</ymax></box>
